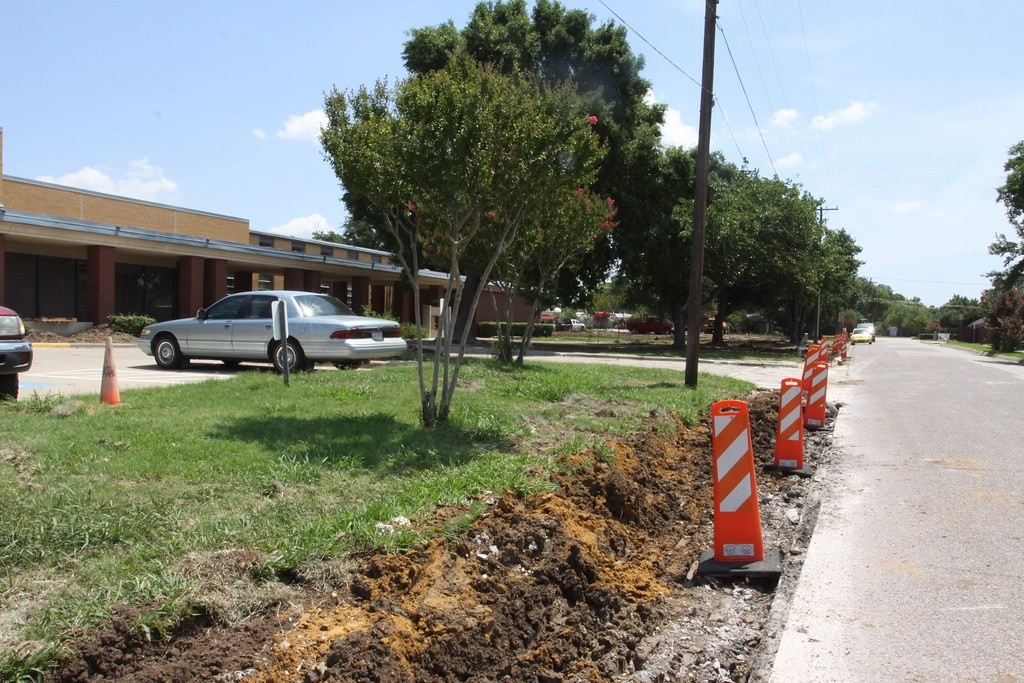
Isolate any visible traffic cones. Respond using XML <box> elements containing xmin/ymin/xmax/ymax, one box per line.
<box><xmin>100</xmin><ymin>337</ymin><xmax>126</xmax><ymax>408</ymax></box>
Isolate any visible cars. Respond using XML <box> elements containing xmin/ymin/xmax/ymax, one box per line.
<box><xmin>850</xmin><ymin>322</ymin><xmax>876</xmax><ymax>345</ymax></box>
<box><xmin>136</xmin><ymin>290</ymin><xmax>407</xmax><ymax>374</ymax></box>
<box><xmin>0</xmin><ymin>306</ymin><xmax>33</xmax><ymax>401</ymax></box>
<box><xmin>704</xmin><ymin>314</ymin><xmax>775</xmax><ymax>334</ymax></box>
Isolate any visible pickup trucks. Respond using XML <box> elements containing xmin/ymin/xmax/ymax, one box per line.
<box><xmin>556</xmin><ymin>319</ymin><xmax>585</xmax><ymax>331</ymax></box>
<box><xmin>627</xmin><ymin>314</ymin><xmax>674</xmax><ymax>334</ymax></box>
